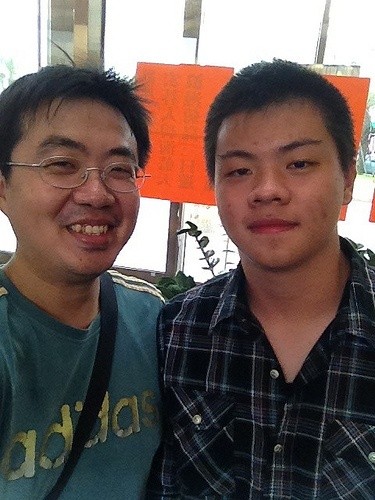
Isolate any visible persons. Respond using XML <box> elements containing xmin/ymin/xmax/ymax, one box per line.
<box><xmin>0</xmin><ymin>65</ymin><xmax>170</xmax><ymax>500</ymax></box>
<box><xmin>155</xmin><ymin>55</ymin><xmax>375</xmax><ymax>499</ymax></box>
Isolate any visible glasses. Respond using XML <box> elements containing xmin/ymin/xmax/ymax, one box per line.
<box><xmin>4</xmin><ymin>155</ymin><xmax>153</xmax><ymax>193</ymax></box>
